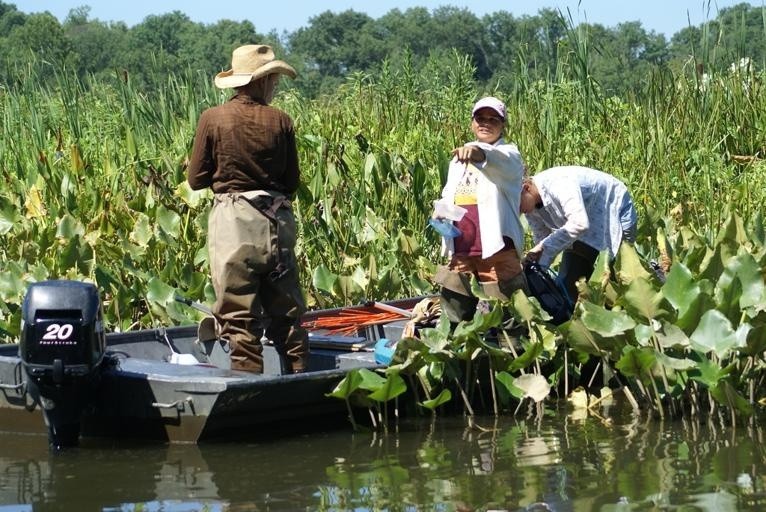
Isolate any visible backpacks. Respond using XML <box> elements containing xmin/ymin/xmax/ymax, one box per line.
<box><xmin>523</xmin><ymin>258</ymin><xmax>573</xmax><ymax>325</ymax></box>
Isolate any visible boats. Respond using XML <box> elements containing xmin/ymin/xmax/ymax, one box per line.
<box><xmin>2</xmin><ymin>274</ymin><xmax>500</xmax><ymax>442</ymax></box>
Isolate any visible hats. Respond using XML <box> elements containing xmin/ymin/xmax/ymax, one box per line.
<box><xmin>214</xmin><ymin>44</ymin><xmax>298</xmax><ymax>88</ymax></box>
<box><xmin>472</xmin><ymin>97</ymin><xmax>507</xmax><ymax>119</ymax></box>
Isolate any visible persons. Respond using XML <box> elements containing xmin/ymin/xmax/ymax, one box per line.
<box><xmin>187</xmin><ymin>44</ymin><xmax>309</xmax><ymax>374</ymax></box>
<box><xmin>517</xmin><ymin>166</ymin><xmax>638</xmax><ymax>297</ymax></box>
<box><xmin>432</xmin><ymin>97</ymin><xmax>530</xmax><ymax>333</ymax></box>
